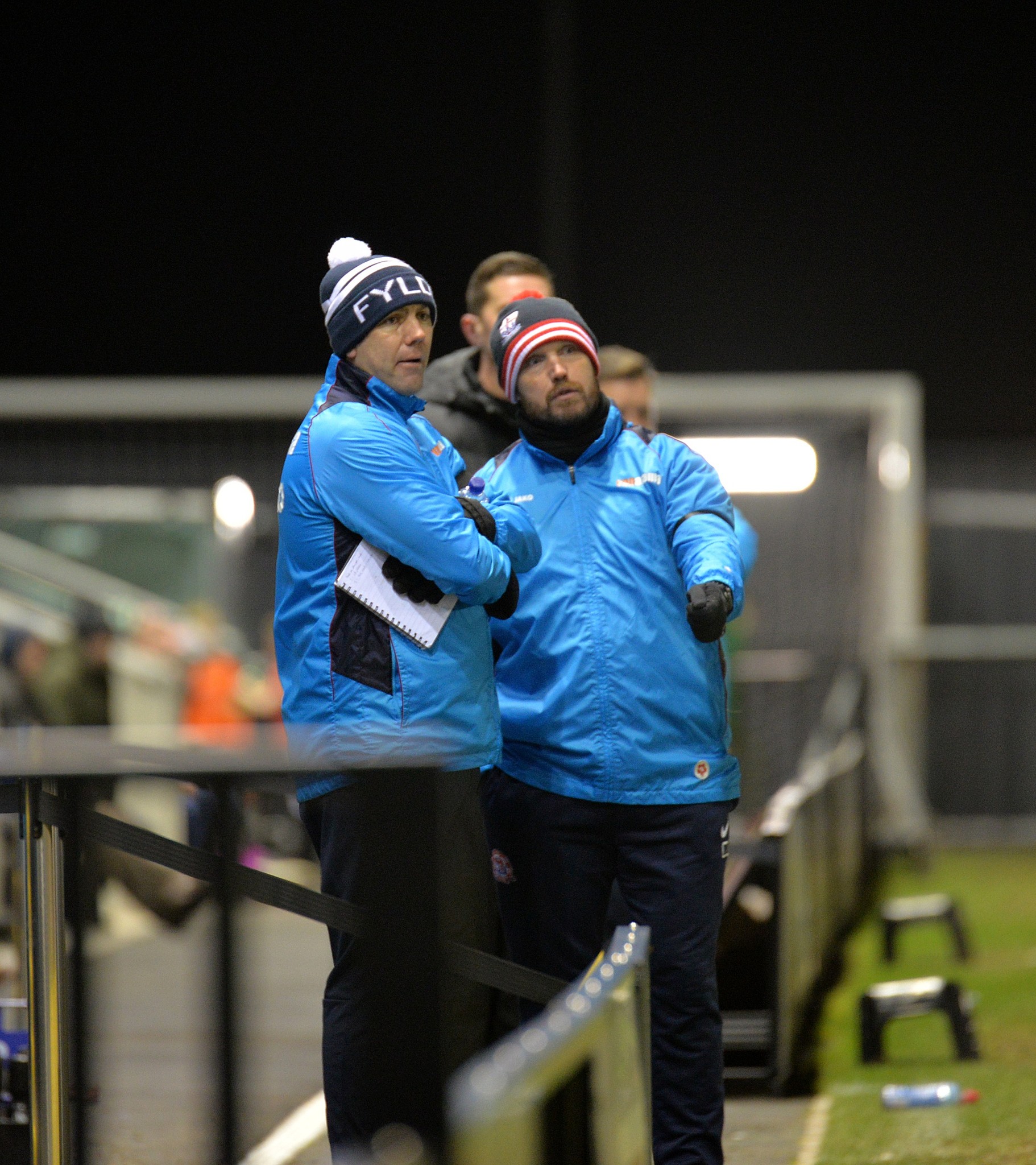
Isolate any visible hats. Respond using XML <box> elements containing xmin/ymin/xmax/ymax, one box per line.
<box><xmin>319</xmin><ymin>235</ymin><xmax>438</xmax><ymax>358</ymax></box>
<box><xmin>489</xmin><ymin>291</ymin><xmax>602</xmax><ymax>405</ymax></box>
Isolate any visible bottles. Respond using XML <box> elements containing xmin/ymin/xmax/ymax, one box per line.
<box><xmin>879</xmin><ymin>1081</ymin><xmax>980</xmax><ymax>1108</ymax></box>
<box><xmin>458</xmin><ymin>477</ymin><xmax>489</xmax><ymax>504</ymax></box>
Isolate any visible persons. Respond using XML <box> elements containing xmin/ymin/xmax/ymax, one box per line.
<box><xmin>443</xmin><ymin>283</ymin><xmax>747</xmax><ymax>1165</ymax></box>
<box><xmin>587</xmin><ymin>343</ymin><xmax>764</xmax><ymax>626</ymax></box>
<box><xmin>0</xmin><ymin>599</ymin><xmax>294</xmax><ymax>982</ymax></box>
<box><xmin>269</xmin><ymin>234</ymin><xmax>525</xmax><ymax>1165</ymax></box>
<box><xmin>396</xmin><ymin>241</ymin><xmax>585</xmax><ymax>485</ymax></box>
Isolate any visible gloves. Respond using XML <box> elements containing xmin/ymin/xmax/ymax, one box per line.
<box><xmin>382</xmin><ymin>496</ymin><xmax>497</xmax><ymax>604</ymax></box>
<box><xmin>684</xmin><ymin>581</ymin><xmax>731</xmax><ymax>644</ymax></box>
<box><xmin>484</xmin><ymin>568</ymin><xmax>520</xmax><ymax>621</ymax></box>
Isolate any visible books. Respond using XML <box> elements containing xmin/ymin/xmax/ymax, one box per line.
<box><xmin>333</xmin><ymin>536</ymin><xmax>460</xmax><ymax>654</ymax></box>
